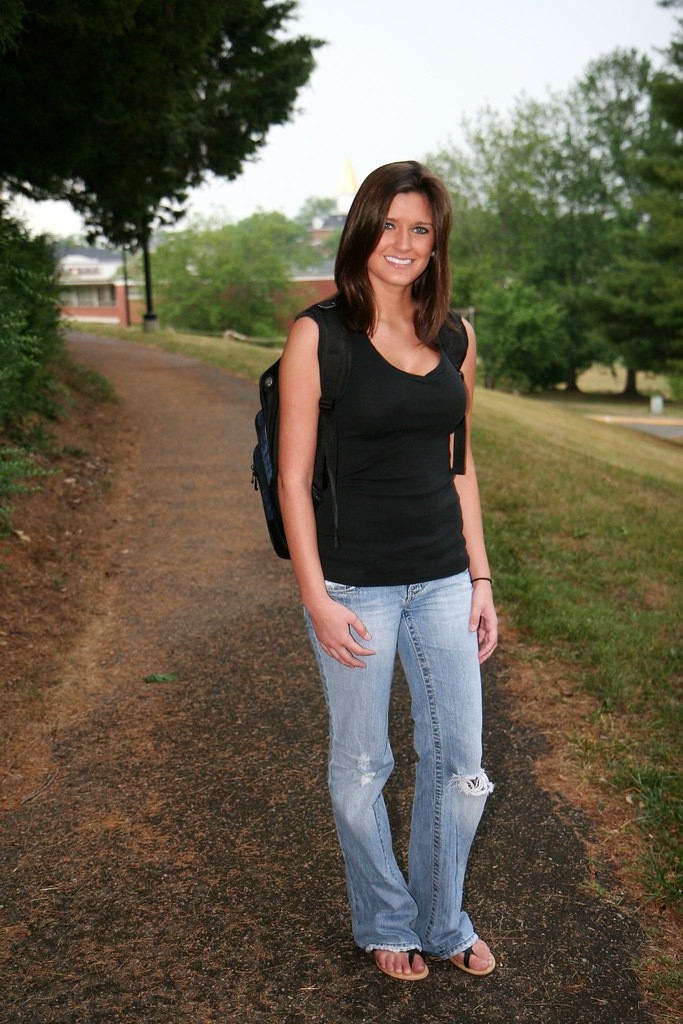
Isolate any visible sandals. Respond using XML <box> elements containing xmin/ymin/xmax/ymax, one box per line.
<box><xmin>450</xmin><ymin>940</ymin><xmax>496</xmax><ymax>976</ymax></box>
<box><xmin>373</xmin><ymin>948</ymin><xmax>430</xmax><ymax>980</ymax></box>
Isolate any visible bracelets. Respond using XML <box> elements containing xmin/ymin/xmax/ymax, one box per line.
<box><xmin>471</xmin><ymin>577</ymin><xmax>493</xmax><ymax>586</ymax></box>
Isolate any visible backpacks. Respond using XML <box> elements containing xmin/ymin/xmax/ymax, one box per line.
<box><xmin>250</xmin><ymin>298</ymin><xmax>471</xmax><ymax>561</ymax></box>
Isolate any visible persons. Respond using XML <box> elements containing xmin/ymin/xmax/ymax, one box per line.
<box><xmin>278</xmin><ymin>162</ymin><xmax>499</xmax><ymax>979</ymax></box>
